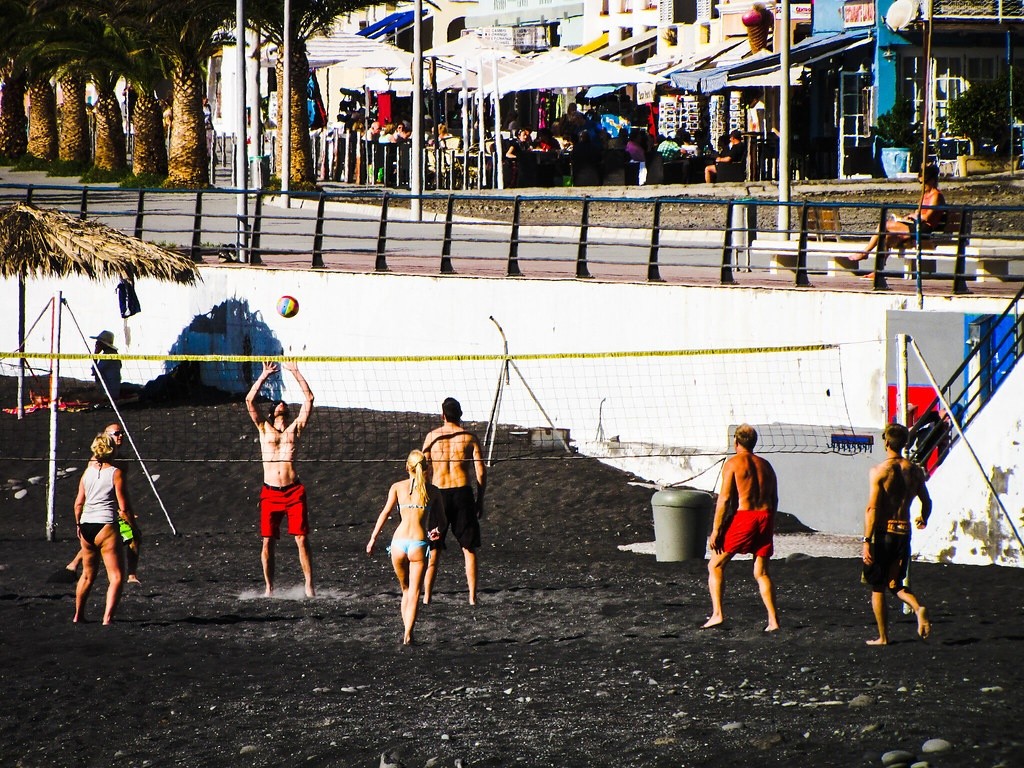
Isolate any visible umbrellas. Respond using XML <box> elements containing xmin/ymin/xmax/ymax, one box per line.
<box><xmin>256</xmin><ymin>31</ymin><xmax>671</xmax><ymax>104</ymax></box>
<box><xmin>0</xmin><ymin>202</ymin><xmax>205</xmax><ymax>420</ymax></box>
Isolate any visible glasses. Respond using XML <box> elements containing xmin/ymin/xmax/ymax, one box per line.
<box><xmin>109</xmin><ymin>431</ymin><xmax>125</xmax><ymax>436</ymax></box>
<box><xmin>882</xmin><ymin>433</ymin><xmax>885</xmax><ymax>439</ymax></box>
<box><xmin>399</xmin><ymin>127</ymin><xmax>405</xmax><ymax>129</ymax></box>
<box><xmin>919</xmin><ymin>180</ymin><xmax>928</xmax><ymax>184</ymax></box>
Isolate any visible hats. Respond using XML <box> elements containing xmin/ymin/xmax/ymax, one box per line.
<box><xmin>949</xmin><ymin>403</ymin><xmax>964</xmax><ymax>428</ymax></box>
<box><xmin>89</xmin><ymin>331</ymin><xmax>118</xmax><ymax>350</ymax></box>
<box><xmin>731</xmin><ymin>130</ymin><xmax>744</xmax><ymax>140</ymax></box>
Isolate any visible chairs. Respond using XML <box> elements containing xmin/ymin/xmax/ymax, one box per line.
<box><xmin>717</xmin><ymin>150</ymin><xmax>748</xmax><ymax>183</ymax></box>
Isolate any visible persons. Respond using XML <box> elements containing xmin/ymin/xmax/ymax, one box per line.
<box><xmin>73</xmin><ymin>434</ymin><xmax>132</xmax><ymax>625</ymax></box>
<box><xmin>200</xmin><ymin>91</ymin><xmax>749</xmax><ymax>185</ymax></box>
<box><xmin>849</xmin><ymin>167</ymin><xmax>945</xmax><ymax>280</ymax></box>
<box><xmin>366</xmin><ymin>450</ymin><xmax>446</xmax><ymax>646</ymax></box>
<box><xmin>860</xmin><ymin>423</ymin><xmax>932</xmax><ymax>645</ymax></box>
<box><xmin>422</xmin><ymin>398</ymin><xmax>486</xmax><ymax>607</ymax></box>
<box><xmin>245</xmin><ymin>359</ymin><xmax>315</xmax><ymax>598</ymax></box>
<box><xmin>65</xmin><ymin>422</ymin><xmax>142</xmax><ymax>585</ymax></box>
<box><xmin>701</xmin><ymin>425</ymin><xmax>780</xmax><ymax>632</ymax></box>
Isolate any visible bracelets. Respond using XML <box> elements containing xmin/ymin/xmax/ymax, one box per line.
<box><xmin>371</xmin><ymin>535</ymin><xmax>376</xmax><ymax>540</ymax></box>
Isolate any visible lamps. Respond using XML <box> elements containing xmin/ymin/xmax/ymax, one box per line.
<box><xmin>856</xmin><ymin>56</ymin><xmax>874</xmax><ymax>79</ymax></box>
<box><xmin>796</xmin><ymin>68</ymin><xmax>813</xmax><ymax>81</ymax></box>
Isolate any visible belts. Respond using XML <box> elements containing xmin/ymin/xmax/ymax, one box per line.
<box><xmin>263</xmin><ymin>479</ymin><xmax>301</xmax><ymax>492</ymax></box>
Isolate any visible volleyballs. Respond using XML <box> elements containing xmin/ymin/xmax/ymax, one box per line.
<box><xmin>277</xmin><ymin>295</ymin><xmax>300</xmax><ymax>319</ymax></box>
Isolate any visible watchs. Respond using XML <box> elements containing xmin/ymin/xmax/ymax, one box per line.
<box><xmin>861</xmin><ymin>537</ymin><xmax>871</xmax><ymax>543</ymax></box>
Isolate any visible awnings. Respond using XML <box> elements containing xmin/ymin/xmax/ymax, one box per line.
<box><xmin>672</xmin><ymin>31</ymin><xmax>872</xmax><ymax>93</ymax></box>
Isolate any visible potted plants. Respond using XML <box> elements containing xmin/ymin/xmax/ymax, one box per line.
<box><xmin>865</xmin><ymin>98</ymin><xmax>921</xmax><ymax>180</ymax></box>
<box><xmin>948</xmin><ymin>76</ymin><xmax>1024</xmax><ymax>177</ymax></box>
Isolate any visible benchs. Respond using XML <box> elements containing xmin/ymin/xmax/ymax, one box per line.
<box><xmin>796</xmin><ymin>205</ymin><xmax>873</xmax><ymax>243</ymax></box>
<box><xmin>889</xmin><ymin>210</ymin><xmax>1024</xmax><ymax>284</ymax></box>
<box><xmin>751</xmin><ymin>239</ymin><xmax>876</xmax><ymax>278</ymax></box>
